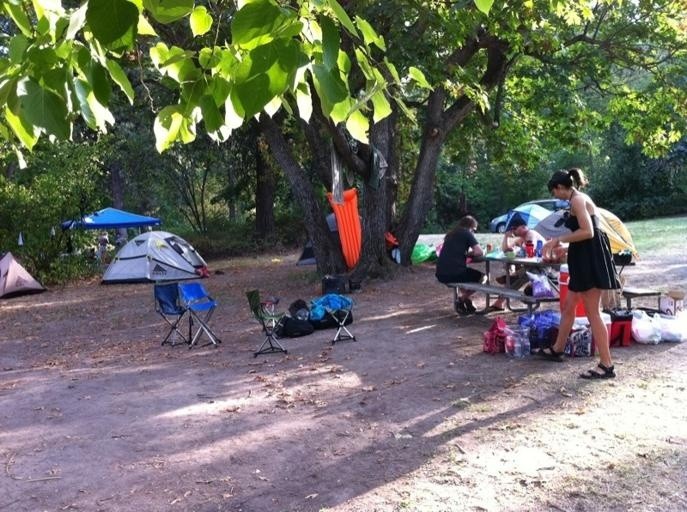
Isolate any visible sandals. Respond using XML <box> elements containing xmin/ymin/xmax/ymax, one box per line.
<box><xmin>580</xmin><ymin>363</ymin><xmax>616</xmax><ymax>378</ymax></box>
<box><xmin>530</xmin><ymin>347</ymin><xmax>565</xmax><ymax>362</ymax></box>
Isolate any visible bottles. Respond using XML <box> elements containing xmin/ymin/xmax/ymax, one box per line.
<box><xmin>526</xmin><ymin>240</ymin><xmax>534</xmax><ymax>258</ymax></box>
<box><xmin>535</xmin><ymin>240</ymin><xmax>544</xmax><ymax>258</ymax></box>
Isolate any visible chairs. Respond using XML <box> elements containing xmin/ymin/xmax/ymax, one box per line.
<box><xmin>152</xmin><ymin>280</ymin><xmax>358</xmax><ymax>358</ymax></box>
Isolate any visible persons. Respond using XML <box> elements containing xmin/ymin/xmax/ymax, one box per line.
<box><xmin>99</xmin><ymin>231</ymin><xmax>109</xmax><ymax>264</ymax></box>
<box><xmin>529</xmin><ymin>167</ymin><xmax>616</xmax><ymax>379</ymax></box>
<box><xmin>483</xmin><ymin>218</ymin><xmax>547</xmax><ymax>311</ymax></box>
<box><xmin>434</xmin><ymin>215</ymin><xmax>488</xmax><ymax>314</ymax></box>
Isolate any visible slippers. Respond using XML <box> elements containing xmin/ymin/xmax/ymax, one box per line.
<box><xmin>498</xmin><ymin>276</ymin><xmax>515</xmax><ymax>283</ymax></box>
<box><xmin>487</xmin><ymin>306</ymin><xmax>504</xmax><ymax>312</ymax></box>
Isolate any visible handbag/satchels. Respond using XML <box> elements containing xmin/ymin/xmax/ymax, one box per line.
<box><xmin>483</xmin><ymin>318</ymin><xmax>513</xmax><ymax>353</ymax></box>
<box><xmin>276</xmin><ymin>316</ymin><xmax>314</xmax><ymax>338</ymax></box>
<box><xmin>308</xmin><ymin>309</ymin><xmax>352</xmax><ymax>330</ymax></box>
<box><xmin>532</xmin><ymin>280</ymin><xmax>555</xmax><ymax>297</ymax></box>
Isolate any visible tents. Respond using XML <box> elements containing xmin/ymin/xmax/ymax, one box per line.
<box><xmin>294</xmin><ymin>212</ymin><xmax>339</xmax><ymax>266</ymax></box>
<box><xmin>0</xmin><ymin>252</ymin><xmax>45</xmax><ymax>298</ymax></box>
<box><xmin>503</xmin><ymin>203</ymin><xmax>550</xmax><ymax>234</ymax></box>
<box><xmin>535</xmin><ymin>208</ymin><xmax>638</xmax><ymax>260</ymax></box>
<box><xmin>101</xmin><ymin>229</ymin><xmax>208</xmax><ymax>282</ymax></box>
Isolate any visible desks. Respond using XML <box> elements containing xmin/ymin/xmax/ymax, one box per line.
<box><xmin>448</xmin><ymin>246</ymin><xmax>662</xmax><ymax>318</ymax></box>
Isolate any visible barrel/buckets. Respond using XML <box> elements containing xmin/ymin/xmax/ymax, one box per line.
<box><xmin>557</xmin><ymin>262</ymin><xmax>599</xmax><ymax>315</ymax></box>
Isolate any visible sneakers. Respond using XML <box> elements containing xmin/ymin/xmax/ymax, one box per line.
<box><xmin>456</xmin><ymin>298</ymin><xmax>476</xmax><ymax>314</ymax></box>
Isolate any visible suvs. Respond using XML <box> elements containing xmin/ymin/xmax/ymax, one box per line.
<box><xmin>489</xmin><ymin>198</ymin><xmax>569</xmax><ymax>234</ymax></box>
